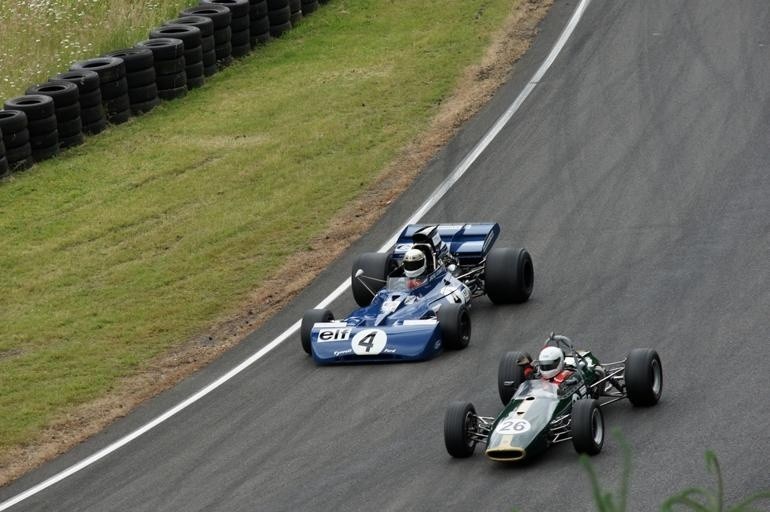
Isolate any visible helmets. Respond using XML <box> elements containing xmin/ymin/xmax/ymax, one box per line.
<box><xmin>402</xmin><ymin>248</ymin><xmax>428</xmax><ymax>278</ymax></box>
<box><xmin>537</xmin><ymin>345</ymin><xmax>567</xmax><ymax>379</ymax></box>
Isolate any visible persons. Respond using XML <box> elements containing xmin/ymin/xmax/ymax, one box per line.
<box><xmin>402</xmin><ymin>249</ymin><xmax>429</xmax><ymax>289</ymax></box>
<box><xmin>537</xmin><ymin>346</ymin><xmax>575</xmax><ymax>387</ymax></box>
